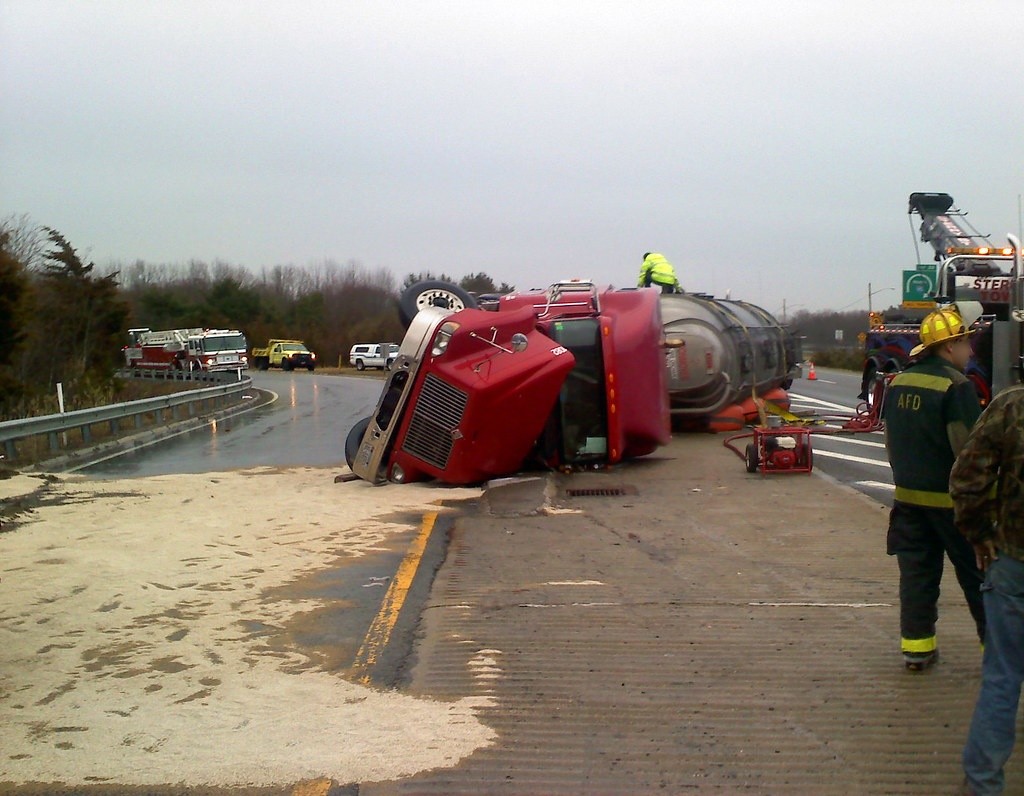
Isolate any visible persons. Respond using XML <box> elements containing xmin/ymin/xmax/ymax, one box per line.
<box><xmin>637</xmin><ymin>253</ymin><xmax>683</xmax><ymax>294</ymax></box>
<box><xmin>948</xmin><ymin>384</ymin><xmax>1024</xmax><ymax>796</ymax></box>
<box><xmin>882</xmin><ymin>310</ymin><xmax>987</xmax><ymax>672</ymax></box>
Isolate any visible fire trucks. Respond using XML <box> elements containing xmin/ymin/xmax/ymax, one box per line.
<box><xmin>121</xmin><ymin>327</ymin><xmax>249</xmax><ymax>374</ymax></box>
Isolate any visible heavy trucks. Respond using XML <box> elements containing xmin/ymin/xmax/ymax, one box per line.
<box><xmin>339</xmin><ymin>281</ymin><xmax>802</xmax><ymax>491</ymax></box>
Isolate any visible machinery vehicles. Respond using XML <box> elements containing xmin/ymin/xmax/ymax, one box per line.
<box><xmin>857</xmin><ymin>190</ymin><xmax>1024</xmax><ymax>419</ymax></box>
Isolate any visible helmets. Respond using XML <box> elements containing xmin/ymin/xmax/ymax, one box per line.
<box><xmin>909</xmin><ymin>310</ymin><xmax>976</xmax><ymax>357</ymax></box>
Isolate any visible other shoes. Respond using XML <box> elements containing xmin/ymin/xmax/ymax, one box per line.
<box><xmin>905</xmin><ymin>647</ymin><xmax>939</xmax><ymax>670</ymax></box>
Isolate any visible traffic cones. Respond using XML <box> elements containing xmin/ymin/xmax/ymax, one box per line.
<box><xmin>805</xmin><ymin>362</ymin><xmax>818</xmax><ymax>380</ymax></box>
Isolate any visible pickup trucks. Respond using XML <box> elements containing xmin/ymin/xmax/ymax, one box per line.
<box><xmin>252</xmin><ymin>339</ymin><xmax>316</xmax><ymax>372</ymax></box>
<box><xmin>349</xmin><ymin>343</ymin><xmax>401</xmax><ymax>371</ymax></box>
